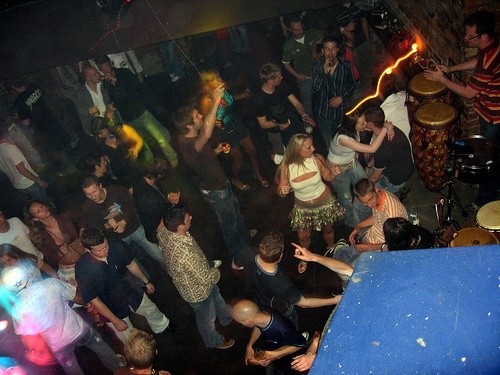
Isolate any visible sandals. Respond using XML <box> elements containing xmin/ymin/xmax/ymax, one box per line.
<box><xmin>298</xmin><ymin>261</ymin><xmax>308</xmax><ymax>273</ymax></box>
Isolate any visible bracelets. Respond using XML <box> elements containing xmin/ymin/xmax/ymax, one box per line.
<box><xmin>383</xmin><ymin>126</ymin><xmax>389</xmax><ymax>131</ymax></box>
<box><xmin>301</xmin><ymin>112</ymin><xmax>308</xmax><ymax>120</ymax></box>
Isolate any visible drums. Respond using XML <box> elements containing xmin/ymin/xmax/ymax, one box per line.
<box><xmin>446</xmin><ymin>225</ymin><xmax>499</xmax><ymax>249</ymax></box>
<box><xmin>408</xmin><ymin>96</ymin><xmax>457</xmax><ymax>191</ymax></box>
<box><xmin>475</xmin><ymin>199</ymin><xmax>500</xmax><ymax>232</ymax></box>
<box><xmin>446</xmin><ymin>134</ymin><xmax>495</xmax><ymax>188</ymax></box>
<box><xmin>406</xmin><ymin>70</ymin><xmax>452</xmax><ymax>104</ymax></box>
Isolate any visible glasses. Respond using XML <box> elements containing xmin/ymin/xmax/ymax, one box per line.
<box><xmin>464</xmin><ymin>32</ymin><xmax>481</xmax><ymax>40</ymax></box>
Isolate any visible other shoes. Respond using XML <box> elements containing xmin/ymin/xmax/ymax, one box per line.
<box><xmin>116</xmin><ymin>354</ymin><xmax>127</xmax><ymax>368</ymax></box>
<box><xmin>166</xmin><ymin>321</ymin><xmax>178</xmax><ymax>332</ymax></box>
<box><xmin>301</xmin><ymin>332</ymin><xmax>309</xmax><ymax>341</ymax></box>
<box><xmin>213</xmin><ymin>259</ymin><xmax>222</xmax><ymax>268</ymax></box>
<box><xmin>232</xmin><ymin>179</ymin><xmax>249</xmax><ymax>191</ymax></box>
<box><xmin>170</xmin><ymin>160</ymin><xmax>179</xmax><ymax>168</ymax></box>
<box><xmin>250</xmin><ymin>229</ymin><xmax>257</xmax><ymax>239</ymax></box>
<box><xmin>256</xmin><ymin>176</ymin><xmax>268</xmax><ymax>187</ymax></box>
<box><xmin>466</xmin><ymin>205</ymin><xmax>478</xmax><ymax>214</ymax></box>
<box><xmin>93</xmin><ymin>313</ymin><xmax>104</xmax><ymax>328</ymax></box>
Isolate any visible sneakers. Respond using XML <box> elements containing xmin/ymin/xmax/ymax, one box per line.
<box><xmin>324</xmin><ymin>239</ymin><xmax>346</xmax><ymax>257</ymax></box>
<box><xmin>216</xmin><ymin>336</ymin><xmax>235</xmax><ymax>349</ymax></box>
<box><xmin>331</xmin><ymin>288</ymin><xmax>344</xmax><ymax>296</ymax></box>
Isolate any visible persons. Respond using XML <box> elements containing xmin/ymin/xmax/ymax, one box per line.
<box><xmin>424</xmin><ymin>9</ymin><xmax>500</xmax><ymax>213</ymax></box>
<box><xmin>0</xmin><ymin>9</ymin><xmax>431</xmax><ymax>375</ymax></box>
